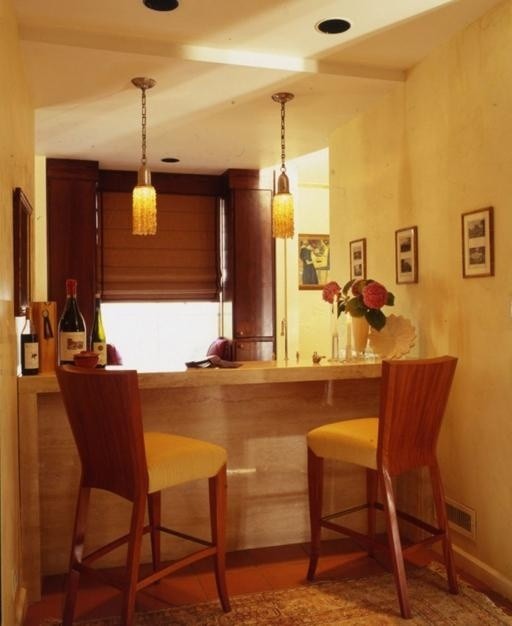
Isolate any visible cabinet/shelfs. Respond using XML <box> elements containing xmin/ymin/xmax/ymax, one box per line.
<box><xmin>217</xmin><ymin>164</ymin><xmax>278</xmax><ymax>341</ymax></box>
<box><xmin>221</xmin><ymin>337</ymin><xmax>279</xmax><ymax>361</ymax></box>
<box><xmin>44</xmin><ymin>156</ymin><xmax>106</xmax><ymax>355</ymax></box>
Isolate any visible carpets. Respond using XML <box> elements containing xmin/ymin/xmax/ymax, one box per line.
<box><xmin>46</xmin><ymin>562</ymin><xmax>512</xmax><ymax>626</ymax></box>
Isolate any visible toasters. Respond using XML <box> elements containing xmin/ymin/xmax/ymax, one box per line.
<box><xmin>91</xmin><ymin>294</ymin><xmax>109</xmax><ymax>367</ymax></box>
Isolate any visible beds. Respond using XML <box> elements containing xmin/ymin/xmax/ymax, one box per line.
<box><xmin>300</xmin><ymin>354</ymin><xmax>465</xmax><ymax>621</ymax></box>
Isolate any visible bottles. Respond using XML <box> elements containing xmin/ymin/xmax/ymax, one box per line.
<box><xmin>22</xmin><ymin>308</ymin><xmax>41</xmax><ymax>374</ymax></box>
<box><xmin>56</xmin><ymin>277</ymin><xmax>87</xmax><ymax>362</ymax></box>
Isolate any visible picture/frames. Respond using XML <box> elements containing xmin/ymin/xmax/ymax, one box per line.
<box><xmin>462</xmin><ymin>205</ymin><xmax>495</xmax><ymax>278</ymax></box>
<box><xmin>13</xmin><ymin>186</ymin><xmax>34</xmax><ymax>318</ymax></box>
<box><xmin>298</xmin><ymin>233</ymin><xmax>329</xmax><ymax>291</ymax></box>
<box><xmin>395</xmin><ymin>226</ymin><xmax>419</xmax><ymax>286</ymax></box>
<box><xmin>349</xmin><ymin>237</ymin><xmax>367</xmax><ymax>283</ymax></box>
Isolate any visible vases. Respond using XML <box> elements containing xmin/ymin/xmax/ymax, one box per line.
<box><xmin>348</xmin><ymin>314</ymin><xmax>369</xmax><ymax>358</ymax></box>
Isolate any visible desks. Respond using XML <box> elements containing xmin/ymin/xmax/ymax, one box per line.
<box><xmin>17</xmin><ymin>357</ymin><xmax>387</xmax><ymax>609</ymax></box>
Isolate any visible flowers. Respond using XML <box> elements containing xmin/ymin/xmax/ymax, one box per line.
<box><xmin>321</xmin><ymin>277</ymin><xmax>397</xmax><ymax>333</ymax></box>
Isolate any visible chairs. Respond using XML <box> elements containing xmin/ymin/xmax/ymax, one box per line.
<box><xmin>52</xmin><ymin>357</ymin><xmax>236</xmax><ymax>625</ymax></box>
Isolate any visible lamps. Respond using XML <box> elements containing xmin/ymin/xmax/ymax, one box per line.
<box><xmin>270</xmin><ymin>91</ymin><xmax>294</xmax><ymax>239</ymax></box>
<box><xmin>131</xmin><ymin>76</ymin><xmax>157</xmax><ymax>235</ymax></box>
